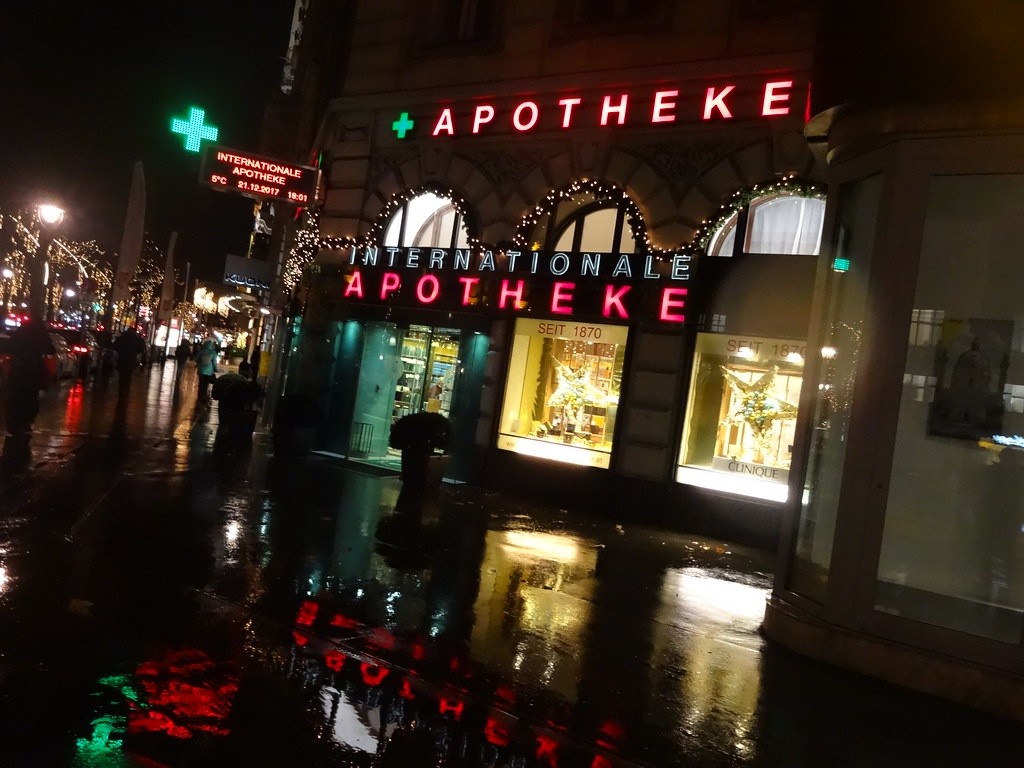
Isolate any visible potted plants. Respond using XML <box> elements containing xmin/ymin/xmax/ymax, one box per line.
<box><xmin>390</xmin><ymin>412</ymin><xmax>459</xmax><ymax>517</ymax></box>
<box><xmin>212</xmin><ymin>373</ymin><xmax>260</xmax><ymax>459</ymax></box>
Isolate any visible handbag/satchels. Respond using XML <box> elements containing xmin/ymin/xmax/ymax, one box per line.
<box><xmin>211</xmin><ymin>375</ymin><xmax>217</xmax><ymax>383</ymax></box>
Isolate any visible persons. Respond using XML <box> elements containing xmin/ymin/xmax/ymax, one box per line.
<box><xmin>175</xmin><ymin>338</ymin><xmax>268</xmax><ymax>485</ymax></box>
<box><xmin>426</xmin><ymin>377</ymin><xmax>445</xmax><ymax>401</ymax></box>
<box><xmin>101</xmin><ymin>326</ymin><xmax>166</xmax><ymax>399</ymax></box>
<box><xmin>0</xmin><ymin>304</ymin><xmax>50</xmax><ymax>464</ymax></box>
<box><xmin>564</xmin><ymin>395</ymin><xmax>578</xmax><ymax>444</ymax></box>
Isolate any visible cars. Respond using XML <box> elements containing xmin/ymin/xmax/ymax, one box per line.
<box><xmin>93</xmin><ymin>329</ymin><xmax>161</xmax><ymax>370</ymax></box>
<box><xmin>9</xmin><ymin>329</ymin><xmax>80</xmax><ymax>383</ymax></box>
<box><xmin>57</xmin><ymin>329</ymin><xmax>103</xmax><ymax>376</ymax></box>
